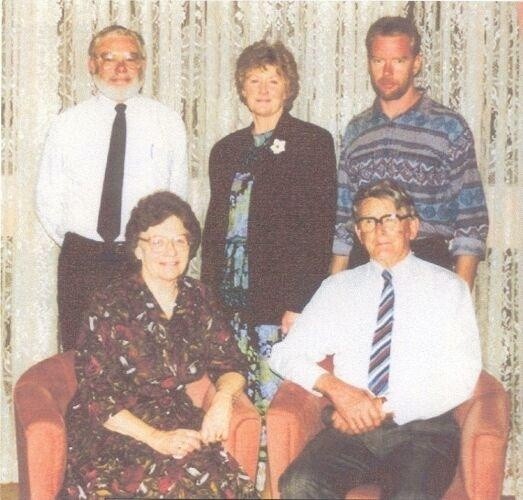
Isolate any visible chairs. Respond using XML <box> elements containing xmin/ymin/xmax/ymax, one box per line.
<box><xmin>13</xmin><ymin>350</ymin><xmax>263</xmax><ymax>499</ymax></box>
<box><xmin>264</xmin><ymin>355</ymin><xmax>510</xmax><ymax>499</ymax></box>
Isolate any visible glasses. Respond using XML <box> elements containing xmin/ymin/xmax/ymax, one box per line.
<box><xmin>356</xmin><ymin>214</ymin><xmax>414</xmax><ymax>233</ymax></box>
<box><xmin>138</xmin><ymin>237</ymin><xmax>194</xmax><ymax>254</ymax></box>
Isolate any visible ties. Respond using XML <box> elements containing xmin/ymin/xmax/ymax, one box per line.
<box><xmin>97</xmin><ymin>104</ymin><xmax>126</xmax><ymax>242</ymax></box>
<box><xmin>368</xmin><ymin>270</ymin><xmax>394</xmax><ymax>396</ymax></box>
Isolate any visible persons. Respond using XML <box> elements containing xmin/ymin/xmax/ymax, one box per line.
<box><xmin>34</xmin><ymin>25</ymin><xmax>190</xmax><ymax>354</ymax></box>
<box><xmin>268</xmin><ymin>179</ymin><xmax>482</xmax><ymax>499</ymax></box>
<box><xmin>52</xmin><ymin>194</ymin><xmax>265</xmax><ymax>500</ymax></box>
<box><xmin>201</xmin><ymin>40</ymin><xmax>338</xmax><ymax>499</ymax></box>
<box><xmin>330</xmin><ymin>15</ymin><xmax>489</xmax><ymax>292</ymax></box>
